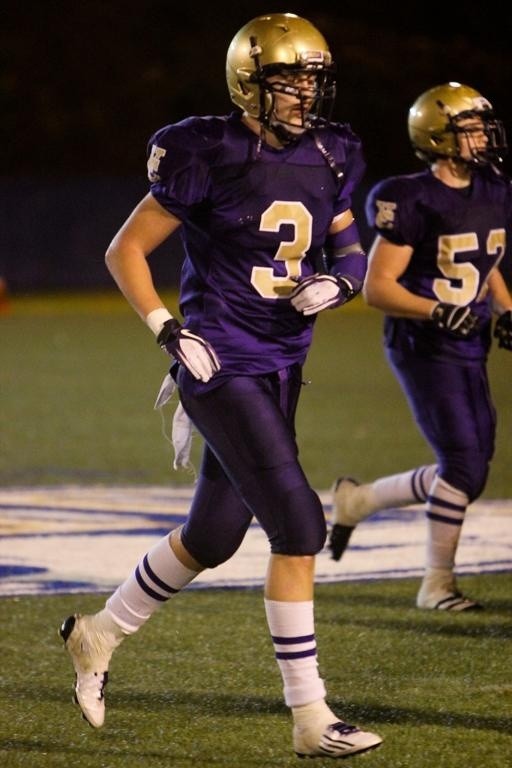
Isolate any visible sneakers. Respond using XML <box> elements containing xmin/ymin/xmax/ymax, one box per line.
<box><xmin>59</xmin><ymin>613</ymin><xmax>109</xmax><ymax>728</ymax></box>
<box><xmin>416</xmin><ymin>591</ymin><xmax>484</xmax><ymax>613</ymax></box>
<box><xmin>291</xmin><ymin>715</ymin><xmax>382</xmax><ymax>759</ymax></box>
<box><xmin>327</xmin><ymin>478</ymin><xmax>360</xmax><ymax>561</ymax></box>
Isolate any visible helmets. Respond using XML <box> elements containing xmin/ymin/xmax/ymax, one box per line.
<box><xmin>226</xmin><ymin>11</ymin><xmax>339</xmax><ymax>142</ymax></box>
<box><xmin>408</xmin><ymin>83</ymin><xmax>507</xmax><ymax>171</ymax></box>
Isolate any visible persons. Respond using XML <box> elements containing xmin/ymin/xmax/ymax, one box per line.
<box><xmin>58</xmin><ymin>8</ymin><xmax>385</xmax><ymax>760</ymax></box>
<box><xmin>325</xmin><ymin>81</ymin><xmax>510</xmax><ymax>613</ymax></box>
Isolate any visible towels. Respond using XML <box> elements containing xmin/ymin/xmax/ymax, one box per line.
<box><xmin>154</xmin><ymin>374</ymin><xmax>192</xmax><ymax>472</ymax></box>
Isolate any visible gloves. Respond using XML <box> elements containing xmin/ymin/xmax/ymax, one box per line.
<box><xmin>289</xmin><ymin>273</ymin><xmax>356</xmax><ymax>316</ymax></box>
<box><xmin>432</xmin><ymin>303</ymin><xmax>479</xmax><ymax>337</ymax></box>
<box><xmin>155</xmin><ymin>320</ymin><xmax>221</xmax><ymax>382</ymax></box>
<box><xmin>493</xmin><ymin>310</ymin><xmax>510</xmax><ymax>349</ymax></box>
